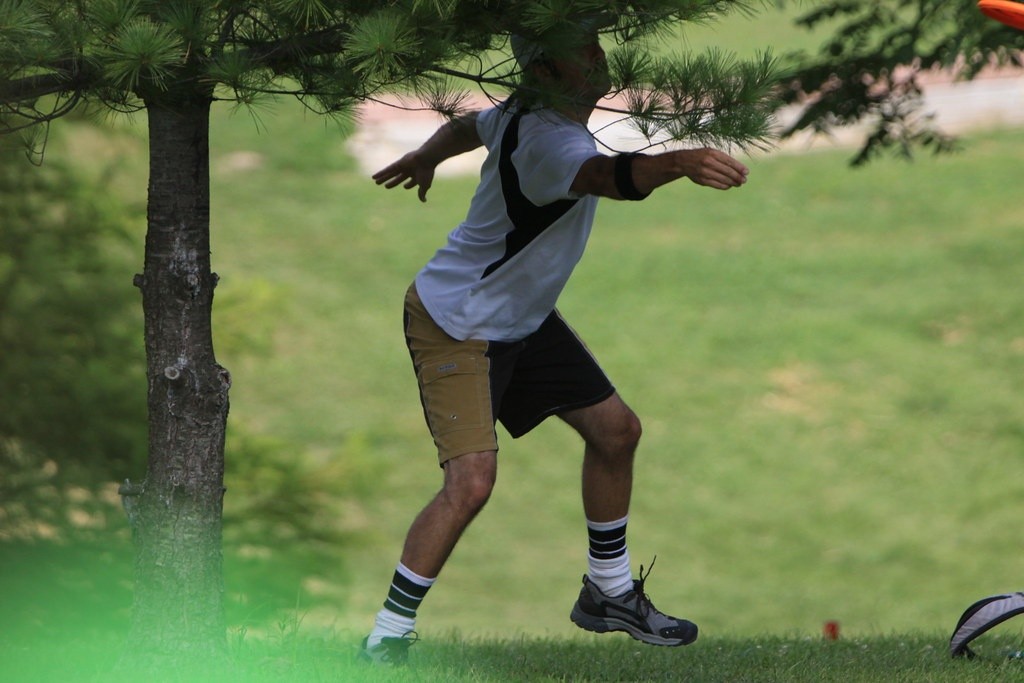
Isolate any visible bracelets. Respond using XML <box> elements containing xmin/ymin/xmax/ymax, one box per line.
<box><xmin>614</xmin><ymin>151</ymin><xmax>655</xmax><ymax>200</ymax></box>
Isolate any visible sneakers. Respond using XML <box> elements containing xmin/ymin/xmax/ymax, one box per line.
<box><xmin>570</xmin><ymin>556</ymin><xmax>698</xmax><ymax>646</ymax></box>
<box><xmin>357</xmin><ymin>630</ymin><xmax>421</xmax><ymax>667</ymax></box>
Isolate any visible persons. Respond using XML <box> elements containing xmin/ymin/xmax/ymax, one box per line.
<box><xmin>359</xmin><ymin>0</ymin><xmax>752</xmax><ymax>679</ymax></box>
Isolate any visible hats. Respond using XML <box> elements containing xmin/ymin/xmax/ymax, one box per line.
<box><xmin>510</xmin><ymin>11</ymin><xmax>620</xmax><ymax>69</ymax></box>
